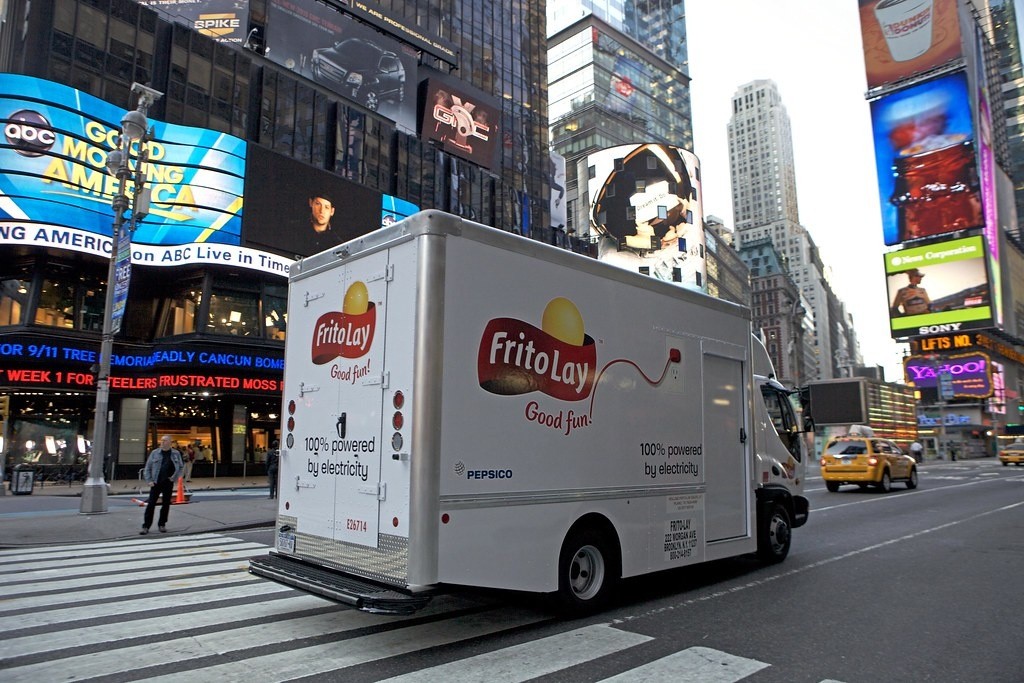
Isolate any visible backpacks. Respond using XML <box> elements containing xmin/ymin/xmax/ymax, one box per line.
<box><xmin>182</xmin><ymin>451</ymin><xmax>189</xmax><ymax>462</ymax></box>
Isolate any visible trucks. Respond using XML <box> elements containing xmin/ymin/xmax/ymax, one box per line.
<box><xmin>246</xmin><ymin>207</ymin><xmax>811</xmax><ymax>618</ymax></box>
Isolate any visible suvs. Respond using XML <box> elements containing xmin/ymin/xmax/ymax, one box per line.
<box><xmin>820</xmin><ymin>434</ymin><xmax>919</xmax><ymax>494</ymax></box>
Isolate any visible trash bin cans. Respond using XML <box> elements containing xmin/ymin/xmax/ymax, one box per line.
<box><xmin>10</xmin><ymin>462</ymin><xmax>35</xmax><ymax>495</ymax></box>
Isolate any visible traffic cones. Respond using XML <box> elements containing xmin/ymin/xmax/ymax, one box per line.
<box><xmin>170</xmin><ymin>477</ymin><xmax>190</xmax><ymax>504</ymax></box>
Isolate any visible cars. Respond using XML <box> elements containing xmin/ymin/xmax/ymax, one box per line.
<box><xmin>998</xmin><ymin>440</ymin><xmax>1024</xmax><ymax>465</ymax></box>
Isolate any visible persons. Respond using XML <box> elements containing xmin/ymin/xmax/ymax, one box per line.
<box><xmin>266</xmin><ymin>440</ymin><xmax>279</xmax><ymax>499</ymax></box>
<box><xmin>254</xmin><ymin>444</ymin><xmax>267</xmax><ymax>462</ymax></box>
<box><xmin>950</xmin><ymin>440</ymin><xmax>956</xmax><ymax>461</ymax></box>
<box><xmin>981</xmin><ymin>444</ymin><xmax>987</xmax><ymax>456</ymax></box>
<box><xmin>284</xmin><ymin>197</ymin><xmax>344</xmax><ymax>255</ymax></box>
<box><xmin>172</xmin><ymin>440</ymin><xmax>212</xmax><ymax>482</ymax></box>
<box><xmin>139</xmin><ymin>435</ymin><xmax>184</xmax><ymax>535</ymax></box>
<box><xmin>959</xmin><ymin>442</ymin><xmax>969</xmax><ymax>459</ymax></box>
<box><xmin>911</xmin><ymin>439</ymin><xmax>923</xmax><ymax>463</ymax></box>
<box><xmin>992</xmin><ymin>438</ymin><xmax>997</xmax><ymax>456</ymax></box>
<box><xmin>892</xmin><ymin>269</ymin><xmax>932</xmax><ymax>317</ymax></box>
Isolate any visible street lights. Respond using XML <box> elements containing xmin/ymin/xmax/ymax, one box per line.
<box><xmin>79</xmin><ymin>95</ymin><xmax>149</xmax><ymax>514</ymax></box>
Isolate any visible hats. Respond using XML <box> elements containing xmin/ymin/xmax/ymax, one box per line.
<box><xmin>906</xmin><ymin>270</ymin><xmax>925</xmax><ymax>278</ymax></box>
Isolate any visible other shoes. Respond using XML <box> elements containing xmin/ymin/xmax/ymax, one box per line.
<box><xmin>158</xmin><ymin>526</ymin><xmax>167</xmax><ymax>532</ymax></box>
<box><xmin>138</xmin><ymin>527</ymin><xmax>150</xmax><ymax>535</ymax></box>
